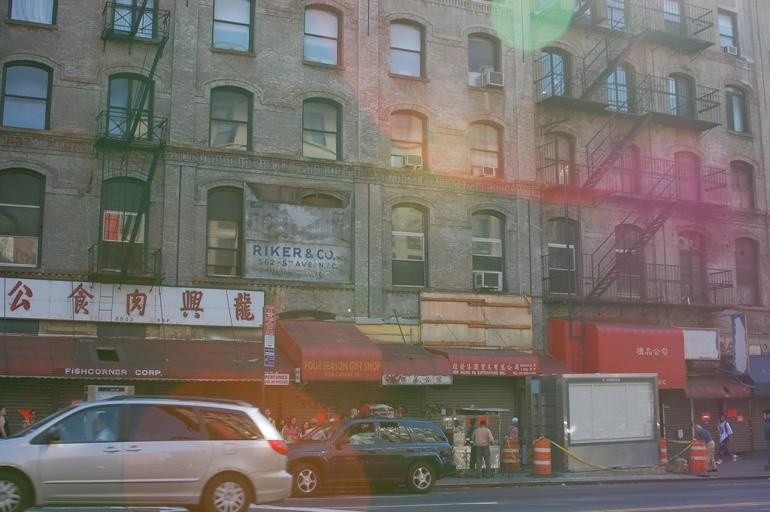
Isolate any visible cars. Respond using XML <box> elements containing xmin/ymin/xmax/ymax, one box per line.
<box><xmin>286</xmin><ymin>418</ymin><xmax>454</xmax><ymax>499</ymax></box>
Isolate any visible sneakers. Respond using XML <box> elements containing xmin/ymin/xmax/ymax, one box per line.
<box><xmin>716</xmin><ymin>459</ymin><xmax>723</xmax><ymax>465</ymax></box>
<box><xmin>732</xmin><ymin>454</ymin><xmax>739</xmax><ymax>463</ymax></box>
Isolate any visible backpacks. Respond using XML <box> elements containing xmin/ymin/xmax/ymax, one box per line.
<box><xmin>724</xmin><ymin>420</ymin><xmax>735</xmax><ymax>435</ymax></box>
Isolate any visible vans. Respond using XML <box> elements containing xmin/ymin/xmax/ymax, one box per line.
<box><xmin>1</xmin><ymin>396</ymin><xmax>293</xmax><ymax>511</ymax></box>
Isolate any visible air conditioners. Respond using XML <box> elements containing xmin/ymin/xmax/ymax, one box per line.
<box><xmin>480</xmin><ymin>164</ymin><xmax>496</xmax><ymax>176</ymax></box>
<box><xmin>723</xmin><ymin>45</ymin><xmax>737</xmax><ymax>56</ymax></box>
<box><xmin>401</xmin><ymin>154</ymin><xmax>423</xmax><ymax>169</ymax></box>
<box><xmin>473</xmin><ymin>271</ymin><xmax>502</xmax><ymax>290</ymax></box>
<box><xmin>481</xmin><ymin>71</ymin><xmax>504</xmax><ymax>89</ymax></box>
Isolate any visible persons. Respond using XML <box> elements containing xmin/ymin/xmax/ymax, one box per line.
<box><xmin>23</xmin><ymin>410</ymin><xmax>38</xmax><ymax>428</ymax></box>
<box><xmin>469</xmin><ymin>418</ymin><xmax>495</xmax><ymax>478</ymax></box>
<box><xmin>508</xmin><ymin>417</ymin><xmax>518</xmax><ymax>439</ymax></box>
<box><xmin>0</xmin><ymin>406</ymin><xmax>9</xmax><ymax>438</ymax></box>
<box><xmin>695</xmin><ymin>415</ymin><xmax>737</xmax><ymax>471</ymax></box>
<box><xmin>264</xmin><ymin>399</ymin><xmax>371</xmax><ymax>443</ymax></box>
